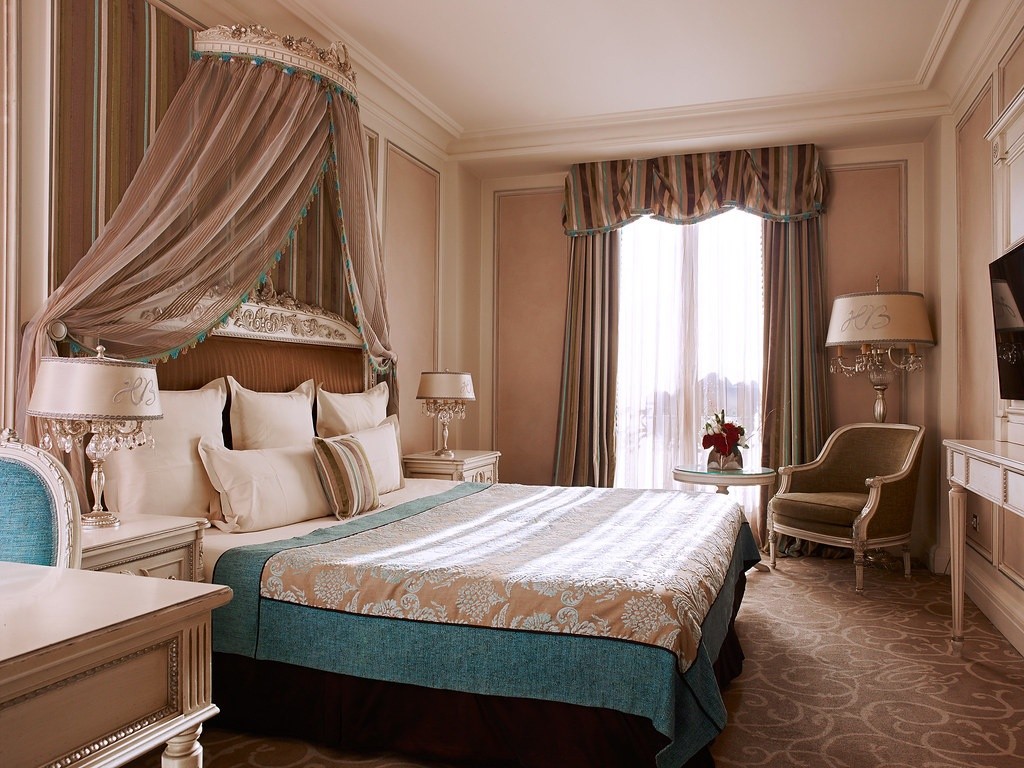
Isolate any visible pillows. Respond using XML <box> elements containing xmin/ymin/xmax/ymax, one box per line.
<box><xmin>312</xmin><ymin>434</ymin><xmax>386</xmax><ymax>522</ymax></box>
<box><xmin>314</xmin><ymin>380</ymin><xmax>389</xmax><ymax>440</ymax></box>
<box><xmin>226</xmin><ymin>374</ymin><xmax>315</xmax><ymax>450</ymax></box>
<box><xmin>198</xmin><ymin>435</ymin><xmax>332</xmax><ymax>534</ymax></box>
<box><xmin>101</xmin><ymin>377</ymin><xmax>227</xmax><ymax>525</ymax></box>
<box><xmin>328</xmin><ymin>413</ymin><xmax>405</xmax><ymax>496</ymax></box>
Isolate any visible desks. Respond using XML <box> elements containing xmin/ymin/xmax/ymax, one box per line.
<box><xmin>0</xmin><ymin>558</ymin><xmax>234</xmax><ymax>768</ymax></box>
<box><xmin>673</xmin><ymin>463</ymin><xmax>776</xmax><ymax>572</ymax></box>
<box><xmin>942</xmin><ymin>438</ymin><xmax>1024</xmax><ymax>657</ymax></box>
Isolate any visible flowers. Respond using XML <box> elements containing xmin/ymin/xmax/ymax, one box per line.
<box><xmin>699</xmin><ymin>409</ymin><xmax>750</xmax><ymax>456</ymax></box>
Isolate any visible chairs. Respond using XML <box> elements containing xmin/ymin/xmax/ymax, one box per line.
<box><xmin>766</xmin><ymin>422</ymin><xmax>925</xmax><ymax>593</ymax></box>
<box><xmin>0</xmin><ymin>428</ymin><xmax>83</xmax><ymax>570</ymax></box>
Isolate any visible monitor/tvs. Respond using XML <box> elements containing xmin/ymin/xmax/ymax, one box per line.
<box><xmin>989</xmin><ymin>242</ymin><xmax>1024</xmax><ymax>401</ymax></box>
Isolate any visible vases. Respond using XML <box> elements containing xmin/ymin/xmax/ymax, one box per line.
<box><xmin>707</xmin><ymin>447</ymin><xmax>744</xmax><ymax>471</ymax></box>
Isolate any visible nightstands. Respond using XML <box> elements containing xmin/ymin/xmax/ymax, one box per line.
<box><xmin>80</xmin><ymin>512</ymin><xmax>211</xmax><ymax>582</ymax></box>
<box><xmin>402</xmin><ymin>448</ymin><xmax>502</xmax><ymax>485</ymax></box>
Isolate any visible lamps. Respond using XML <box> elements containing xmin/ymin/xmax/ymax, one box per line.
<box><xmin>825</xmin><ymin>274</ymin><xmax>935</xmax><ymax>572</ymax></box>
<box><xmin>416</xmin><ymin>369</ymin><xmax>476</xmax><ymax>457</ymax></box>
<box><xmin>26</xmin><ymin>346</ymin><xmax>164</xmax><ymax>528</ymax></box>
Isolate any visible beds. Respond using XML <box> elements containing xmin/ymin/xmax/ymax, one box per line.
<box><xmin>98</xmin><ymin>271</ymin><xmax>762</xmax><ymax>768</ymax></box>
